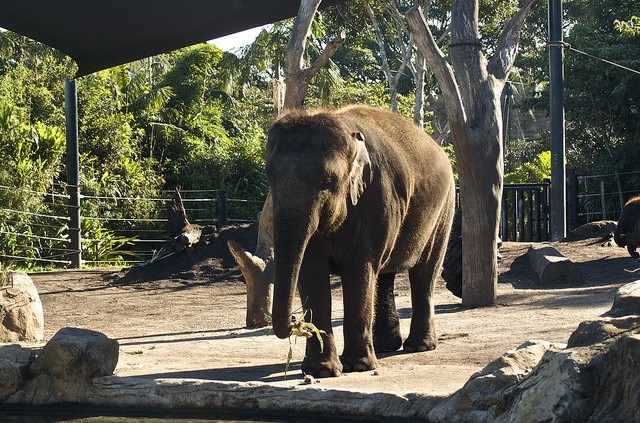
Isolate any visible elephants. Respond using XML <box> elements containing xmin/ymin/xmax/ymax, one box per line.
<box><xmin>265</xmin><ymin>103</ymin><xmax>456</xmax><ymax>379</ymax></box>
<box><xmin>614</xmin><ymin>196</ymin><xmax>640</xmax><ymax>259</ymax></box>
